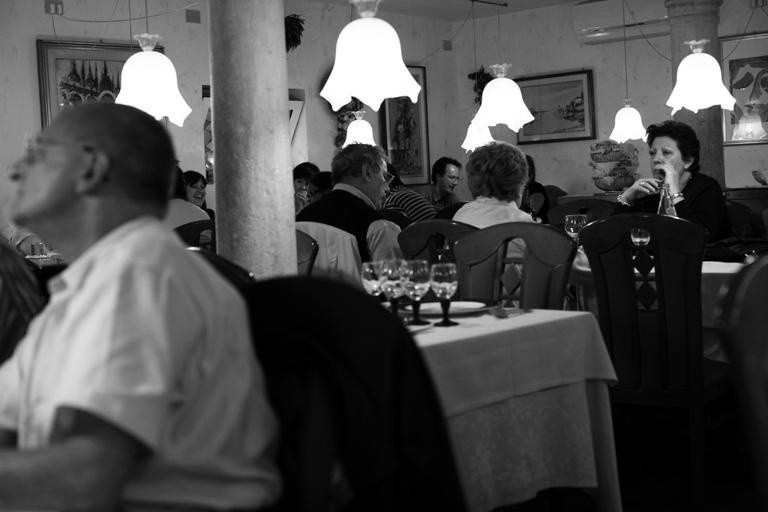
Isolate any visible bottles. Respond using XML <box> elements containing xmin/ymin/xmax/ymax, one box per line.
<box><xmin>657</xmin><ymin>183</ymin><xmax>678</xmax><ymax>218</ymax></box>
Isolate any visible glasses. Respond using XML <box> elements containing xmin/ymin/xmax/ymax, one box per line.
<box><xmin>381</xmin><ymin>169</ymin><xmax>395</xmax><ymax>187</ymax></box>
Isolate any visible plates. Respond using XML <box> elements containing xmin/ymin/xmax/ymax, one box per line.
<box><xmin>403</xmin><ymin>300</ymin><xmax>487</xmax><ymax>316</ymax></box>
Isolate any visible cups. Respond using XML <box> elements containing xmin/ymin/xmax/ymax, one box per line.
<box><xmin>630</xmin><ymin>226</ymin><xmax>651</xmax><ymax>246</ymax></box>
<box><xmin>31</xmin><ymin>241</ymin><xmax>44</xmax><ymax>257</ymax></box>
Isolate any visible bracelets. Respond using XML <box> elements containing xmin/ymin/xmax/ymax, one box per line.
<box><xmin>670</xmin><ymin>192</ymin><xmax>684</xmax><ymax>199</ymax></box>
<box><xmin>617</xmin><ymin>194</ymin><xmax>632</xmax><ymax>206</ymax></box>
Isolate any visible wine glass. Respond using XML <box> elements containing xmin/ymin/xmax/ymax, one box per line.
<box><xmin>360</xmin><ymin>257</ymin><xmax>461</xmax><ymax>330</ymax></box>
<box><xmin>563</xmin><ymin>214</ymin><xmax>587</xmax><ymax>247</ymax></box>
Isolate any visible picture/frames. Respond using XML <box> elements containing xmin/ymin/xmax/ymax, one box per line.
<box><xmin>717</xmin><ymin>30</ymin><xmax>768</xmax><ymax>147</ymax></box>
<box><xmin>34</xmin><ymin>37</ymin><xmax>166</xmax><ymax>135</ymax></box>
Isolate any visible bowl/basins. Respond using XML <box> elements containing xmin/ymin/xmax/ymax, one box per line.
<box><xmin>751</xmin><ymin>169</ymin><xmax>767</xmax><ymax>186</ymax></box>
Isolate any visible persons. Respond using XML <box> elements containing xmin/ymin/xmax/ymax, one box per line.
<box><xmin>395</xmin><ymin>107</ymin><xmax>417</xmax><ymax>175</ymax></box>
<box><xmin>611</xmin><ymin>120</ymin><xmax>733</xmax><ymax>242</ymax></box>
<box><xmin>167</xmin><ymin>164</ymin><xmax>217</xmax><ymax>255</ymax></box>
<box><xmin>293</xmin><ymin>142</ymin><xmax>551</xmax><ymax>302</ymax></box>
<box><xmin>0</xmin><ymin>98</ymin><xmax>285</xmax><ymax>511</ymax></box>
<box><xmin>0</xmin><ymin>235</ymin><xmax>49</xmax><ymax>362</ymax></box>
